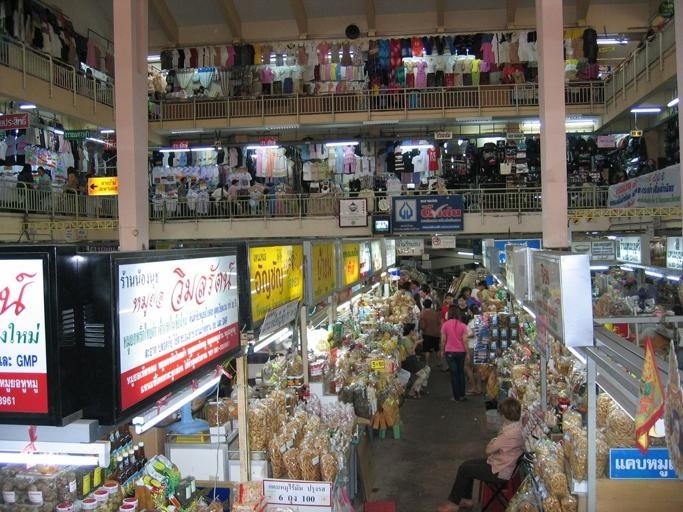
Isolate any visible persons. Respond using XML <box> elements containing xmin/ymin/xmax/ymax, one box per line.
<box><xmin>397</xmin><ymin>264</ymin><xmax>681</xmax><ymax>403</ymax></box>
<box><xmin>567</xmin><ymin>152</ymin><xmax>680</xmax><ymax>209</ymax></box>
<box><xmin>17</xmin><ymin>163</ymin><xmax>541</xmax><ymax>221</ymax></box>
<box><xmin>439</xmin><ymin>397</ymin><xmax>526</xmax><ymax>512</ymax></box>
<box><xmin>55</xmin><ymin>65</ymin><xmax>604</xmax><ymax>119</ymax></box>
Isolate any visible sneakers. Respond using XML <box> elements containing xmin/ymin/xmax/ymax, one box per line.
<box><xmin>452</xmin><ymin>388</ymin><xmax>482</xmax><ymax>403</ymax></box>
<box><xmin>438</xmin><ymin>502</ymin><xmax>473</xmax><ymax>512</ymax></box>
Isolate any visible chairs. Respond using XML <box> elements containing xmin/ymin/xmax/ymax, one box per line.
<box><xmin>481</xmin><ymin>449</ymin><xmax>527</xmax><ymax>512</ymax></box>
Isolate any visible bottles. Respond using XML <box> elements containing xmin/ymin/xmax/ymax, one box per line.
<box><xmin>107</xmin><ymin>424</ymin><xmax>147</xmax><ymax>483</ymax></box>
<box><xmin>56</xmin><ymin>483</ymin><xmax>139</xmax><ymax>512</ymax></box>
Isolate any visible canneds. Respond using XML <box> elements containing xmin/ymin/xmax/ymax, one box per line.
<box><xmin>287</xmin><ymin>373</ymin><xmax>304</xmax><ymax>398</ymax></box>
<box><xmin>204</xmin><ymin>397</ymin><xmax>229</xmax><ymax>428</ymax></box>
